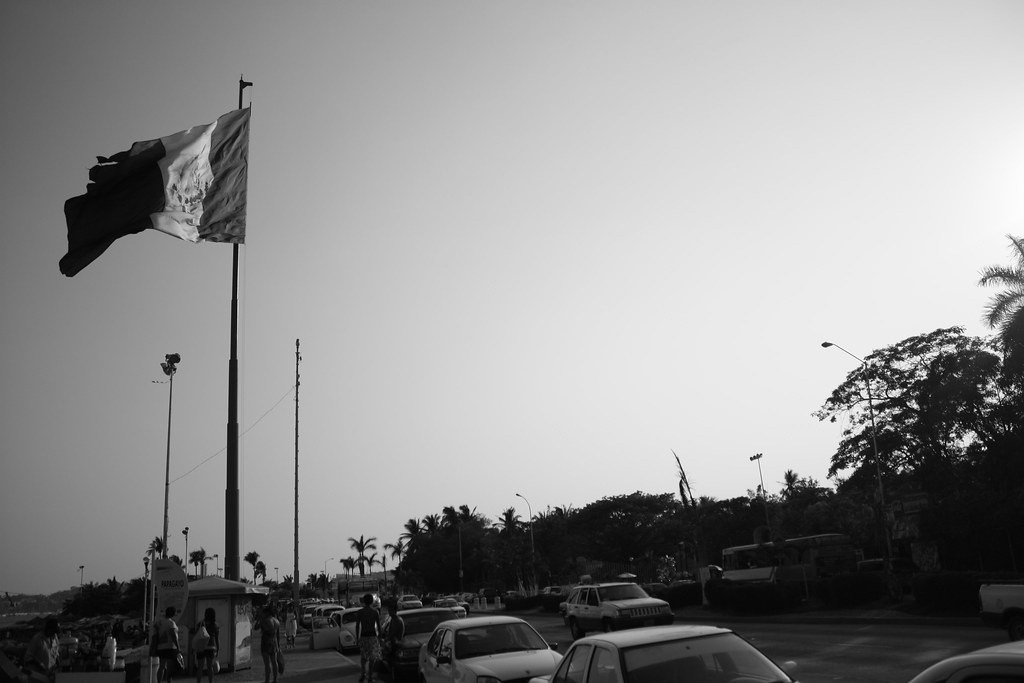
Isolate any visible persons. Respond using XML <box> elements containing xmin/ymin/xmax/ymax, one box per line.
<box><xmin>356</xmin><ymin>592</ymin><xmax>405</xmax><ymax>683</ymax></box>
<box><xmin>408</xmin><ymin>591</ymin><xmax>480</xmax><ymax>608</ymax></box>
<box><xmin>261</xmin><ymin>601</ymin><xmax>306</xmax><ymax>649</ymax></box>
<box><xmin>255</xmin><ymin>608</ymin><xmax>281</xmax><ymax>683</ymax></box>
<box><xmin>148</xmin><ymin>607</ymin><xmax>221</xmax><ymax>683</ymax></box>
<box><xmin>22</xmin><ymin>620</ymin><xmax>60</xmax><ymax>683</ymax></box>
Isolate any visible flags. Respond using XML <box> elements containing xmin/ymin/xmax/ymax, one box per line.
<box><xmin>58</xmin><ymin>107</ymin><xmax>253</xmax><ymax>278</ymax></box>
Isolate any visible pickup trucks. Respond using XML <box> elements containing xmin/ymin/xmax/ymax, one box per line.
<box><xmin>977</xmin><ymin>578</ymin><xmax>1024</xmax><ymax>642</ymax></box>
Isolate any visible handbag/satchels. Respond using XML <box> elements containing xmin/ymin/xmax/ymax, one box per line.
<box><xmin>175</xmin><ymin>654</ymin><xmax>185</xmax><ymax>672</ymax></box>
<box><xmin>191</xmin><ymin>621</ymin><xmax>210</xmax><ymax>653</ymax></box>
<box><xmin>149</xmin><ymin>633</ymin><xmax>160</xmax><ymax>658</ymax></box>
<box><xmin>276</xmin><ymin>652</ymin><xmax>285</xmax><ymax>673</ymax></box>
<box><xmin>214</xmin><ymin>660</ymin><xmax>221</xmax><ymax>673</ymax></box>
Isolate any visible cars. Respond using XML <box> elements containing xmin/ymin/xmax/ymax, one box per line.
<box><xmin>557</xmin><ymin>581</ymin><xmax>675</xmax><ymax>642</ymax></box>
<box><xmin>527</xmin><ymin>622</ymin><xmax>801</xmax><ymax>683</ymax></box>
<box><xmin>906</xmin><ymin>639</ymin><xmax>1024</xmax><ymax>683</ymax></box>
<box><xmin>276</xmin><ymin>591</ymin><xmax>470</xmax><ymax>683</ymax></box>
<box><xmin>416</xmin><ymin>615</ymin><xmax>575</xmax><ymax>683</ymax></box>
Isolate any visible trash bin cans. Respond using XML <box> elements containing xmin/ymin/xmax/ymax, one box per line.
<box><xmin>124</xmin><ymin>654</ymin><xmax>160</xmax><ymax>683</ymax></box>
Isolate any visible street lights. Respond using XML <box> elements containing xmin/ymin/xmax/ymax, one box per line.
<box><xmin>213</xmin><ymin>553</ymin><xmax>218</xmax><ymax>576</ymax></box>
<box><xmin>181</xmin><ymin>526</ymin><xmax>189</xmax><ymax>578</ymax></box>
<box><xmin>516</xmin><ymin>493</ymin><xmax>536</xmax><ymax>597</ymax></box>
<box><xmin>749</xmin><ymin>453</ymin><xmax>774</xmax><ymax>539</ymax></box>
<box><xmin>324</xmin><ymin>557</ymin><xmax>334</xmax><ymax>600</ymax></box>
<box><xmin>159</xmin><ymin>352</ymin><xmax>181</xmax><ymax>557</ymax></box>
<box><xmin>142</xmin><ymin>556</ymin><xmax>150</xmax><ymax>633</ymax></box>
<box><xmin>820</xmin><ymin>341</ymin><xmax>893</xmax><ymax>560</ymax></box>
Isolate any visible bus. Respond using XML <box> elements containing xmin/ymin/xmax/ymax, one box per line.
<box><xmin>721</xmin><ymin>533</ymin><xmax>852</xmax><ymax>588</ymax></box>
